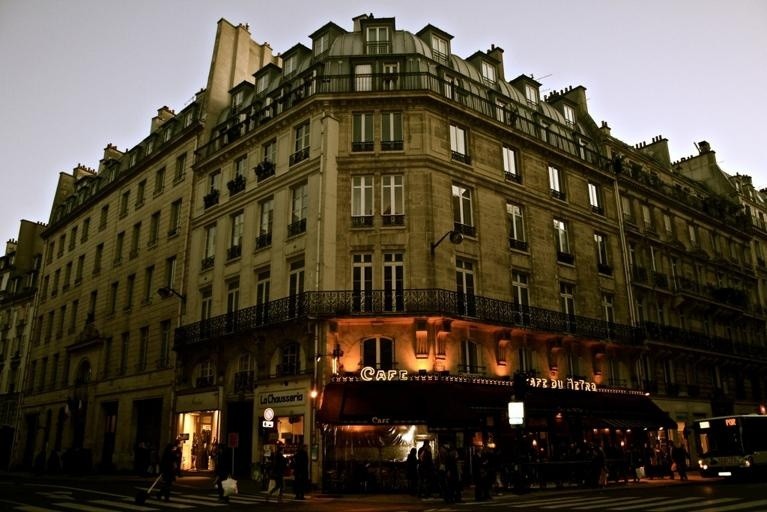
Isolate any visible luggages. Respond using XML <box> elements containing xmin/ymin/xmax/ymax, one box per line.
<box><xmin>133</xmin><ymin>473</ymin><xmax>162</xmax><ymax>505</ymax></box>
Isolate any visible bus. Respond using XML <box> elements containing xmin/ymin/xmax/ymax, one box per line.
<box><xmin>684</xmin><ymin>413</ymin><xmax>767</xmax><ymax>484</ymax></box>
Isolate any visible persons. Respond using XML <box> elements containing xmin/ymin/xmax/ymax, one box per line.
<box><xmin>132</xmin><ymin>433</ymin><xmax>308</xmax><ymax>504</ymax></box>
<box><xmin>403</xmin><ymin>433</ymin><xmax>694</xmax><ymax>505</ymax></box>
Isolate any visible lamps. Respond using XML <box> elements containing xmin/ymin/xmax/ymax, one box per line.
<box><xmin>157</xmin><ymin>286</ymin><xmax>186</xmax><ymax>302</ymax></box>
<box><xmin>431</xmin><ymin>229</ymin><xmax>464</xmax><ymax>257</ymax></box>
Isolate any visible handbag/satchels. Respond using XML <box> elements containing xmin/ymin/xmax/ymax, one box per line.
<box><xmin>221</xmin><ymin>477</ymin><xmax>238</xmax><ymax>496</ymax></box>
